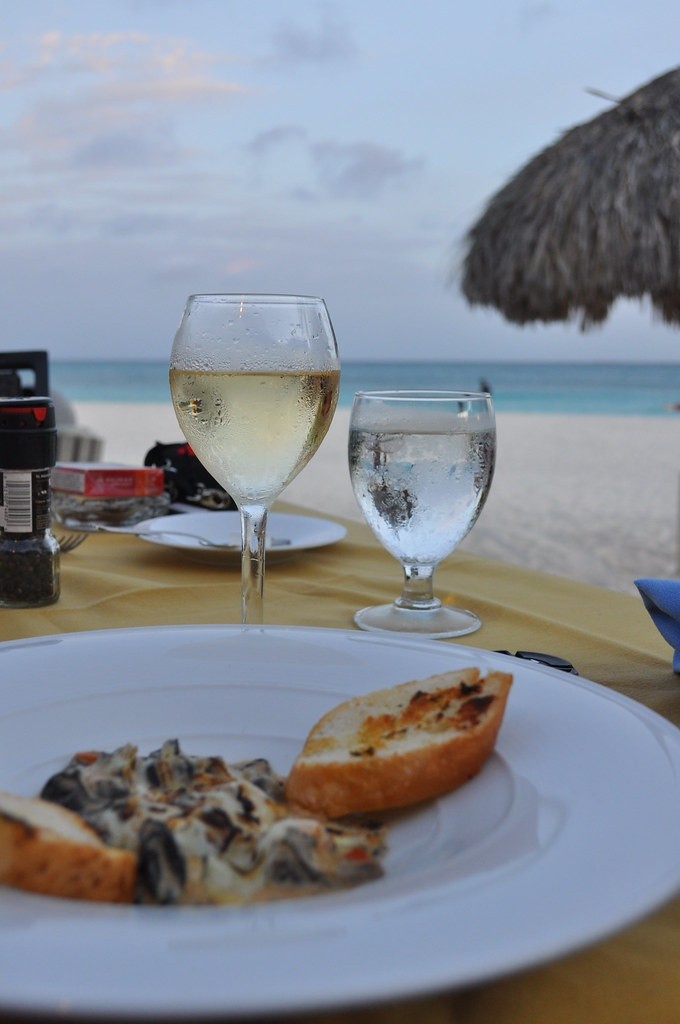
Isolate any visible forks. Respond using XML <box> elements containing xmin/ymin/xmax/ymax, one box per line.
<box><xmin>50</xmin><ymin>534</ymin><xmax>88</xmax><ymax>551</ymax></box>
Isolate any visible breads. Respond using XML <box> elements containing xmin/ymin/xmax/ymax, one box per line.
<box><xmin>0</xmin><ymin>794</ymin><xmax>135</xmax><ymax>905</ymax></box>
<box><xmin>286</xmin><ymin>667</ymin><xmax>514</xmax><ymax>820</ymax></box>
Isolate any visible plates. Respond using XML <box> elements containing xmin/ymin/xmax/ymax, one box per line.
<box><xmin>133</xmin><ymin>511</ymin><xmax>346</xmax><ymax>567</ymax></box>
<box><xmin>0</xmin><ymin>624</ymin><xmax>679</xmax><ymax>1016</ymax></box>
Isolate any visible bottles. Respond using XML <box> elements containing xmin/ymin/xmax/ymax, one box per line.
<box><xmin>0</xmin><ymin>348</ymin><xmax>59</xmax><ymax>609</ymax></box>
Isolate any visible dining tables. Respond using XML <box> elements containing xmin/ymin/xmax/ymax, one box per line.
<box><xmin>0</xmin><ymin>464</ymin><xmax>680</xmax><ymax>1024</ymax></box>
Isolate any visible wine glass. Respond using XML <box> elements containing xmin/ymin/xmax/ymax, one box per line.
<box><xmin>347</xmin><ymin>392</ymin><xmax>498</xmax><ymax>641</ymax></box>
<box><xmin>168</xmin><ymin>293</ymin><xmax>342</xmax><ymax>628</ymax></box>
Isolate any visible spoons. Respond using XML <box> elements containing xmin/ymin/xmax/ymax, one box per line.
<box><xmin>98</xmin><ymin>525</ymin><xmax>292</xmax><ymax>549</ymax></box>
